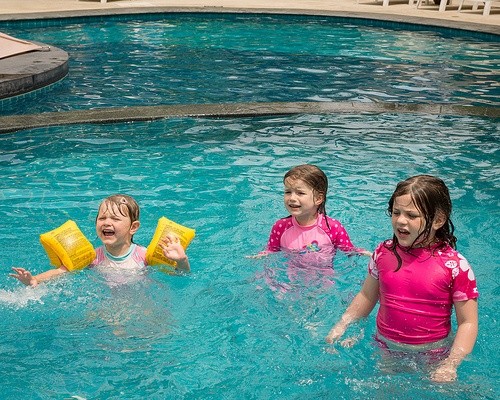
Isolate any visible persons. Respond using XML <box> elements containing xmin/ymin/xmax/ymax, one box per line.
<box><xmin>9</xmin><ymin>193</ymin><xmax>192</xmax><ymax>291</ymax></box>
<box><xmin>323</xmin><ymin>173</ymin><xmax>479</xmax><ymax>382</ymax></box>
<box><xmin>244</xmin><ymin>163</ymin><xmax>372</xmax><ymax>254</ymax></box>
